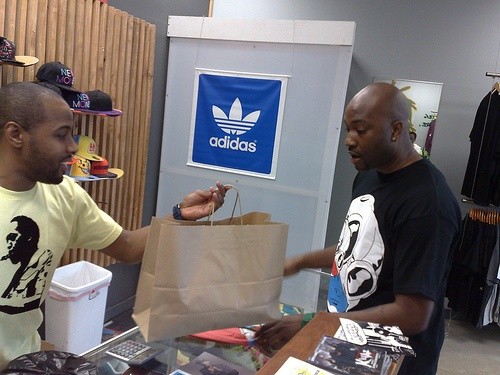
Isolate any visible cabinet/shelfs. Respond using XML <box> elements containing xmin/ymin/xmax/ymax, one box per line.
<box><xmin>0</xmin><ymin>56</ymin><xmax>126</xmax><ymax>183</ymax></box>
<box><xmin>82</xmin><ymin>311</ymin><xmax>406</xmax><ymax>375</ymax></box>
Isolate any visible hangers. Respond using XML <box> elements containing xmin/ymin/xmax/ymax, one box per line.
<box><xmin>468</xmin><ymin>203</ymin><xmax>500</xmax><ymax>228</ymax></box>
<box><xmin>483</xmin><ymin>75</ymin><xmax>500</xmax><ymax>100</ymax></box>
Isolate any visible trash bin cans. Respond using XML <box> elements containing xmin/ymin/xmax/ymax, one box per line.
<box><xmin>45</xmin><ymin>260</ymin><xmax>112</xmax><ymax>355</ymax></box>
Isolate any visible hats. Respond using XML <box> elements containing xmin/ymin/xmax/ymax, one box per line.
<box><xmin>0</xmin><ymin>36</ymin><xmax>25</xmax><ymax>64</ymax></box>
<box><xmin>72</xmin><ymin>89</ymin><xmax>123</xmax><ymax>119</ymax></box>
<box><xmin>36</xmin><ymin>60</ymin><xmax>81</xmax><ymax>95</ymax></box>
<box><xmin>65</xmin><ymin>133</ymin><xmax>119</xmax><ymax>181</ymax></box>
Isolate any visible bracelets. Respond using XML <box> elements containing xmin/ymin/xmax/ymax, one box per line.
<box><xmin>173</xmin><ymin>204</ymin><xmax>183</xmax><ymax>220</ymax></box>
<box><xmin>301</xmin><ymin>312</ymin><xmax>314</xmax><ymax>327</ymax></box>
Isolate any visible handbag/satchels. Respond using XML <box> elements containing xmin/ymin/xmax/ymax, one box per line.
<box><xmin>130</xmin><ymin>184</ymin><xmax>289</xmax><ymax>343</ymax></box>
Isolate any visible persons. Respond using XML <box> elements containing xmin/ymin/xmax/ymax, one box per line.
<box><xmin>254</xmin><ymin>82</ymin><xmax>464</xmax><ymax>375</ymax></box>
<box><xmin>0</xmin><ymin>81</ymin><xmax>233</xmax><ymax>371</ymax></box>
<box><xmin>409</xmin><ymin>132</ymin><xmax>422</xmax><ymax>156</ymax></box>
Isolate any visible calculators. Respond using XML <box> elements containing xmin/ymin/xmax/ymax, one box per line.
<box><xmin>106</xmin><ymin>340</ymin><xmax>164</xmax><ymax>364</ymax></box>
